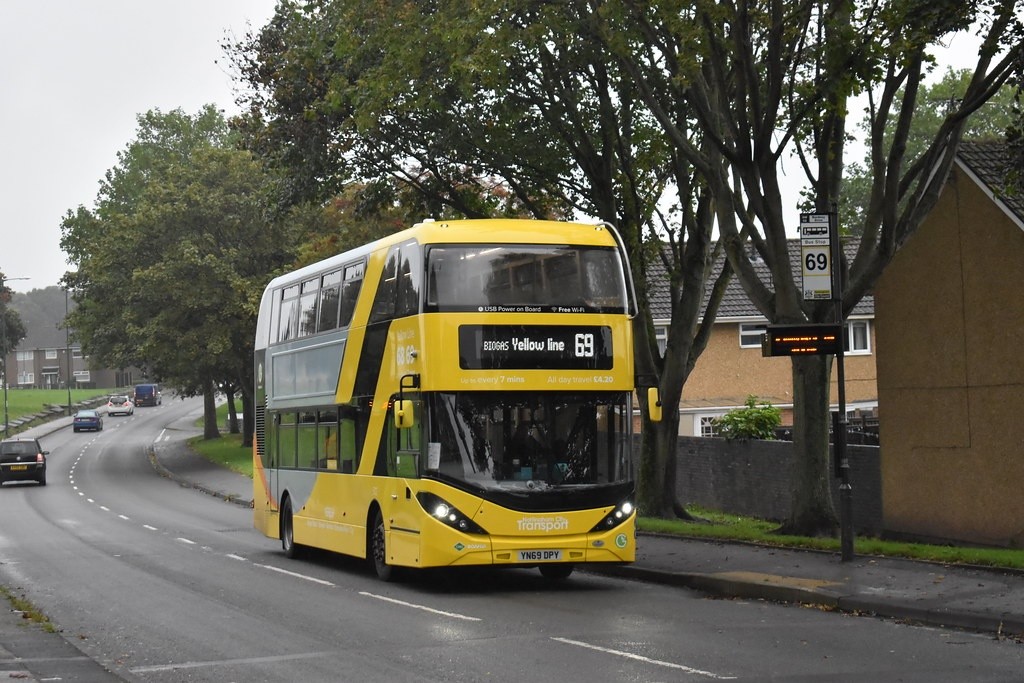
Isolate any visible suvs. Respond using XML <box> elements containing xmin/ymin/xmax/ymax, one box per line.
<box><xmin>107</xmin><ymin>394</ymin><xmax>135</xmax><ymax>416</ymax></box>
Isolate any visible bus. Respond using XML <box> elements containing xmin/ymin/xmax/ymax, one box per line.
<box><xmin>253</xmin><ymin>218</ymin><xmax>663</xmax><ymax>583</ymax></box>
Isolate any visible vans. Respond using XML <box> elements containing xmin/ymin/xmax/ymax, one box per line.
<box><xmin>133</xmin><ymin>383</ymin><xmax>162</xmax><ymax>408</ymax></box>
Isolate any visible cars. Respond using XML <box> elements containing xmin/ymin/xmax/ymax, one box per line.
<box><xmin>0</xmin><ymin>437</ymin><xmax>51</xmax><ymax>487</ymax></box>
<box><xmin>73</xmin><ymin>409</ymin><xmax>104</xmax><ymax>433</ymax></box>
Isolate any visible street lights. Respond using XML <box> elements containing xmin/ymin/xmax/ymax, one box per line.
<box><xmin>1</xmin><ymin>277</ymin><xmax>32</xmax><ymax>437</ymax></box>
